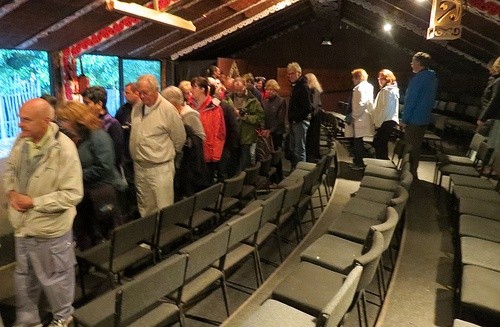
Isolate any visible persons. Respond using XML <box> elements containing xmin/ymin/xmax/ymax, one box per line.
<box><xmin>400</xmin><ymin>52</ymin><xmax>438</xmax><ymax>183</ymax></box>
<box><xmin>42</xmin><ymin>66</ymin><xmax>287</xmax><ymax>251</ymax></box>
<box><xmin>373</xmin><ymin>69</ymin><xmax>400</xmax><ymax>160</ymax></box>
<box><xmin>283</xmin><ymin>62</ymin><xmax>323</xmax><ymax>178</ymax></box>
<box><xmin>4</xmin><ymin>98</ymin><xmax>83</xmax><ymax>327</ymax></box>
<box><xmin>477</xmin><ymin>56</ymin><xmax>500</xmax><ymax>125</ymax></box>
<box><xmin>345</xmin><ymin>69</ymin><xmax>374</xmax><ymax>170</ymax></box>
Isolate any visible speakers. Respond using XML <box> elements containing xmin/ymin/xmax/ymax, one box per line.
<box><xmin>426</xmin><ymin>0</ymin><xmax>462</xmax><ymax>40</ymax></box>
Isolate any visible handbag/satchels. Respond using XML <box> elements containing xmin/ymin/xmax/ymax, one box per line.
<box><xmin>478</xmin><ymin>120</ymin><xmax>495</xmax><ymax>136</ymax></box>
<box><xmin>87</xmin><ymin>184</ymin><xmax>122</xmax><ymax>220</ymax></box>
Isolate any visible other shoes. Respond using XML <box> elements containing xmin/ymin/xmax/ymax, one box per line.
<box><xmin>352</xmin><ymin>167</ymin><xmax>363</xmax><ymax>170</ymax></box>
<box><xmin>48</xmin><ymin>316</ymin><xmax>73</xmax><ymax>327</ymax></box>
<box><xmin>13</xmin><ymin>321</ymin><xmax>43</xmax><ymax>327</ymax></box>
<box><xmin>347</xmin><ymin>164</ymin><xmax>356</xmax><ymax>168</ymax></box>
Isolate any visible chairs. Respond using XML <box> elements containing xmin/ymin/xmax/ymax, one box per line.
<box><xmin>0</xmin><ymin>94</ymin><xmax>500</xmax><ymax>327</ymax></box>
